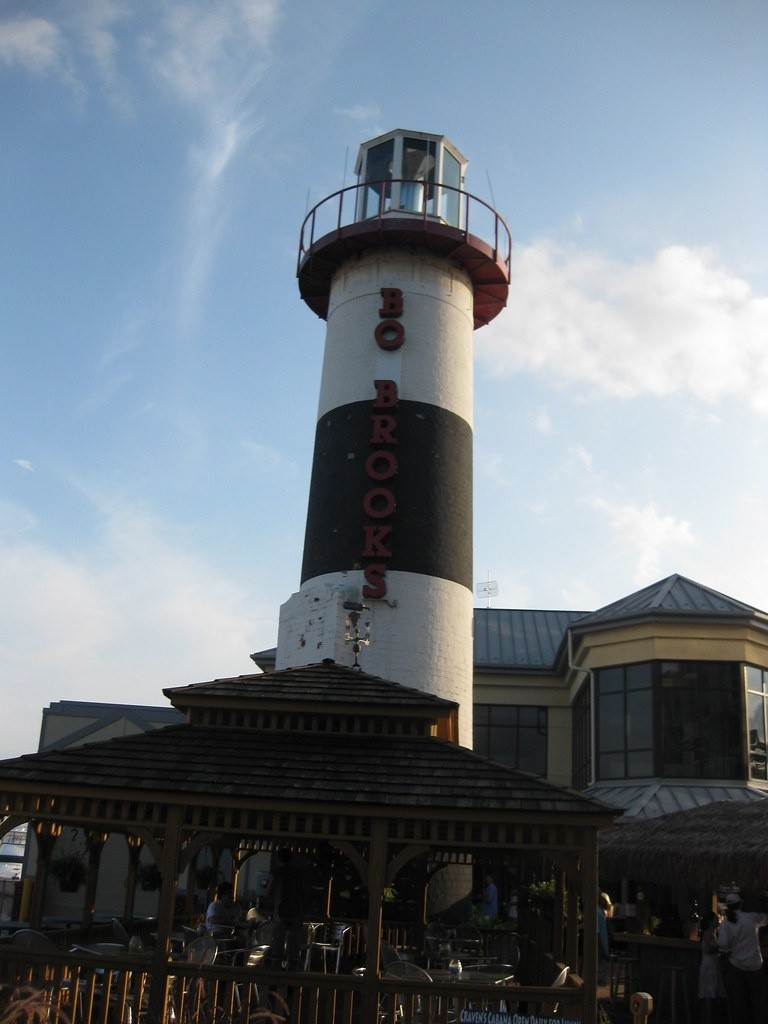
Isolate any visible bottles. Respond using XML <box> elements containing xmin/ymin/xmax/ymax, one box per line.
<box><xmin>449</xmin><ymin>959</ymin><xmax>462</xmax><ymax>982</ymax></box>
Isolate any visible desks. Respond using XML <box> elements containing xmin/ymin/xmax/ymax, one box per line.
<box><xmin>72</xmin><ymin>942</ymin><xmax>186</xmax><ymax>960</ymax></box>
<box><xmin>613</xmin><ymin>933</ymin><xmax>702</xmax><ymax>1024</ymax></box>
<box><xmin>421</xmin><ymin>950</ymin><xmax>499</xmax><ymax>968</ymax></box>
<box><xmin>42</xmin><ymin>917</ymin><xmax>106</xmax><ymax>929</ymax></box>
<box><xmin>425</xmin><ymin>935</ymin><xmax>481</xmax><ymax>947</ymax></box>
<box><xmin>0</xmin><ymin>920</ymin><xmax>49</xmax><ymax>936</ymax></box>
<box><xmin>412</xmin><ymin>969</ymin><xmax>515</xmax><ymax>983</ymax></box>
<box><xmin>212</xmin><ymin>922</ymin><xmax>249</xmax><ymax>940</ymax></box>
<box><xmin>287</xmin><ymin>921</ymin><xmax>326</xmax><ymax>971</ymax></box>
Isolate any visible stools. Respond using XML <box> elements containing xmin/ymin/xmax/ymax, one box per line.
<box><xmin>613</xmin><ymin>956</ymin><xmax>640</xmax><ymax>1013</ymax></box>
<box><xmin>609</xmin><ymin>952</ymin><xmax>635</xmax><ymax>1004</ymax></box>
<box><xmin>654</xmin><ymin>965</ymin><xmax>691</xmax><ymax>1024</ymax></box>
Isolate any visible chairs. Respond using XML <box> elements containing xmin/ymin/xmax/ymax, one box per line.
<box><xmin>0</xmin><ymin>916</ymin><xmax>572</xmax><ymax>1024</ymax></box>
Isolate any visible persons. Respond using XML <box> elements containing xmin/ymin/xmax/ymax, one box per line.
<box><xmin>598</xmin><ymin>887</ymin><xmax>614</xmax><ymax>987</ymax></box>
<box><xmin>697</xmin><ymin>893</ymin><xmax>768</xmax><ymax>1024</ymax></box>
<box><xmin>206</xmin><ymin>847</ymin><xmax>307</xmax><ymax>973</ymax></box>
<box><xmin>505</xmin><ymin>888</ymin><xmax>518</xmax><ymax>922</ymax></box>
<box><xmin>477</xmin><ymin>872</ymin><xmax>497</xmax><ymax>922</ymax></box>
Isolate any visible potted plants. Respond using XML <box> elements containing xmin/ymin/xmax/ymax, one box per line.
<box><xmin>122</xmin><ymin>860</ymin><xmax>163</xmax><ymax>893</ymax></box>
<box><xmin>45</xmin><ymin>845</ymin><xmax>95</xmax><ymax>894</ymax></box>
<box><xmin>526</xmin><ymin>879</ymin><xmax>583</xmax><ymax>928</ymax></box>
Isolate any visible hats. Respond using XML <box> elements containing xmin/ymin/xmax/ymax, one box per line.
<box><xmin>727</xmin><ymin>894</ymin><xmax>743</xmax><ymax>904</ymax></box>
